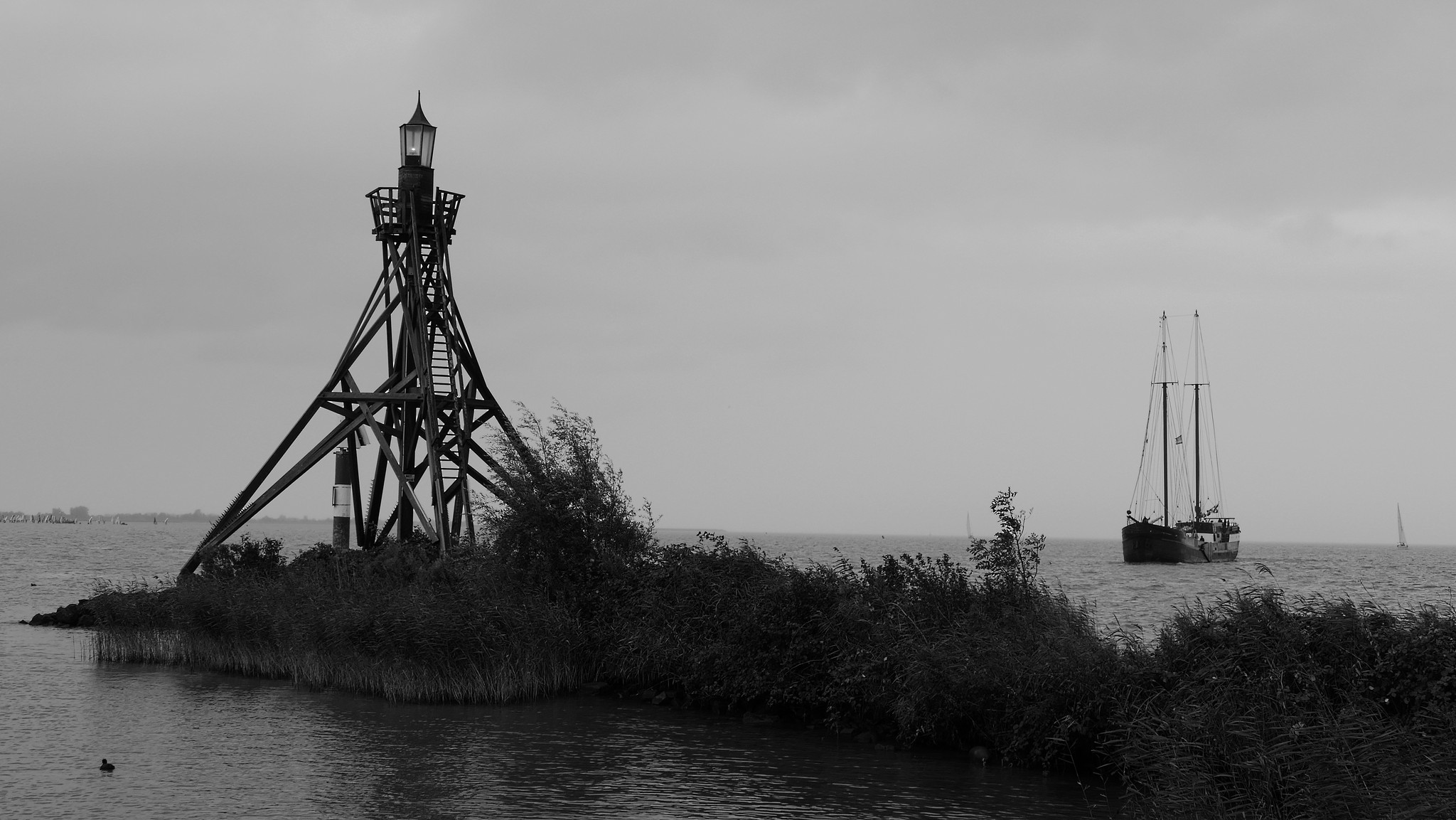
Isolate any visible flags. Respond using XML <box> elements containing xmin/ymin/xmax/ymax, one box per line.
<box><xmin>1175</xmin><ymin>435</ymin><xmax>1183</xmax><ymax>444</ymax></box>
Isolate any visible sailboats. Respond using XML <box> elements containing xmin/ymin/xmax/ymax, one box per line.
<box><xmin>1396</xmin><ymin>503</ymin><xmax>1409</xmax><ymax>548</ymax></box>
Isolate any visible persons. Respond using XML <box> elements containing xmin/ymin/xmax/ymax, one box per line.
<box><xmin>1181</xmin><ymin>518</ymin><xmax>1230</xmax><ymax>542</ymax></box>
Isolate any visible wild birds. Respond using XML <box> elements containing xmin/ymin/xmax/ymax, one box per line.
<box><xmin>100</xmin><ymin>759</ymin><xmax>115</xmax><ymax>771</ymax></box>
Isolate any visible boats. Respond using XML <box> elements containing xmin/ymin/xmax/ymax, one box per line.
<box><xmin>1121</xmin><ymin>310</ymin><xmax>1243</xmax><ymax>565</ymax></box>
